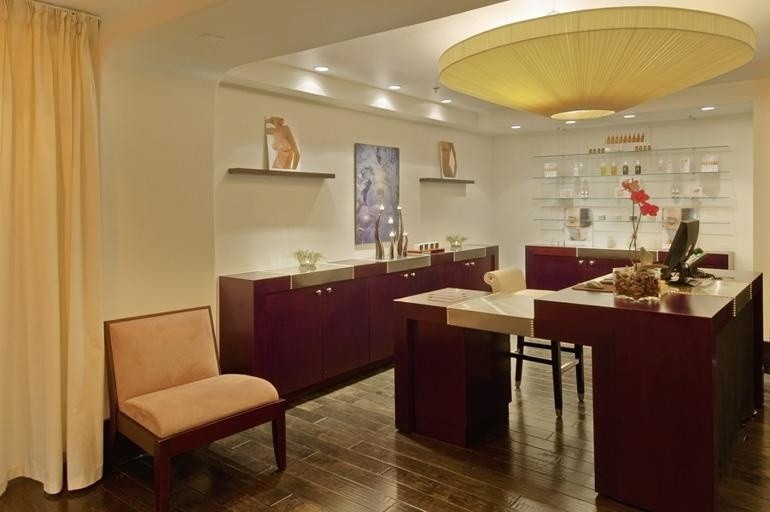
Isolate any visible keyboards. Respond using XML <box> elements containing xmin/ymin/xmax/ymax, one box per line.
<box><xmin>601</xmin><ymin>275</ymin><xmax>614</xmax><ymax>284</ymax></box>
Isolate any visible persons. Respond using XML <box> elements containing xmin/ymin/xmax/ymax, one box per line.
<box><xmin>266</xmin><ymin>118</ymin><xmax>300</xmax><ymax>170</ymax></box>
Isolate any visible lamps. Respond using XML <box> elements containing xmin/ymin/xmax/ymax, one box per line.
<box><xmin>433</xmin><ymin>9</ymin><xmax>756</xmax><ymax>123</ymax></box>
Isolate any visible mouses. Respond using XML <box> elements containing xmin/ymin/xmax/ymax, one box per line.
<box><xmin>584</xmin><ymin>280</ymin><xmax>603</xmax><ymax>289</ymax></box>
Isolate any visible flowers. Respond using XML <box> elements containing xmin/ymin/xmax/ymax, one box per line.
<box><xmin>616</xmin><ymin>174</ymin><xmax>658</xmax><ymax>298</ymax></box>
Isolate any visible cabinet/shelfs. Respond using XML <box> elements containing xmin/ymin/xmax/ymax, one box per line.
<box><xmin>369</xmin><ymin>257</ymin><xmax>432</xmax><ymax>369</ymax></box>
<box><xmin>219</xmin><ymin>264</ymin><xmax>373</xmax><ymax>401</ymax></box>
<box><xmin>526</xmin><ymin>247</ymin><xmax>640</xmax><ymax>302</ymax></box>
<box><xmin>533</xmin><ymin>144</ymin><xmax>735</xmax><ymax>234</ymax></box>
<box><xmin>430</xmin><ymin>247</ymin><xmax>499</xmax><ymax>297</ymax></box>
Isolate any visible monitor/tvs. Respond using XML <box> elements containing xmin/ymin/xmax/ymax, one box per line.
<box><xmin>660</xmin><ymin>219</ymin><xmax>702</xmax><ymax>286</ymax></box>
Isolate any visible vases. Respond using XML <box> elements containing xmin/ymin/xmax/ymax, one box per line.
<box><xmin>613</xmin><ymin>268</ymin><xmax>668</xmax><ymax>305</ymax></box>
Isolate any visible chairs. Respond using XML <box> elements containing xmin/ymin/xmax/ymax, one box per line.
<box><xmin>483</xmin><ymin>267</ymin><xmax>584</xmax><ymax>419</ymax></box>
<box><xmin>103</xmin><ymin>306</ymin><xmax>286</xmax><ymax>510</ymax></box>
